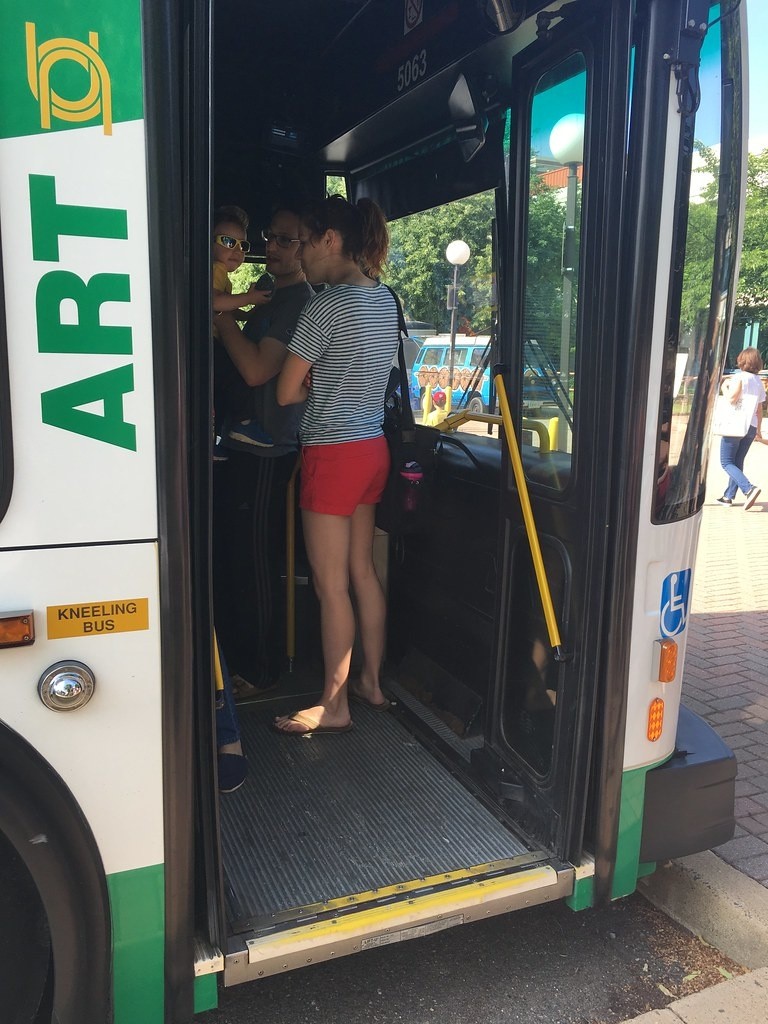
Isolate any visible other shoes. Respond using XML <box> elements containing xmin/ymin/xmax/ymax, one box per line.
<box><xmin>217</xmin><ymin>735</ymin><xmax>248</xmax><ymax>793</ymax></box>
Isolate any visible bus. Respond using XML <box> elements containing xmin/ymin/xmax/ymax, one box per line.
<box><xmin>0</xmin><ymin>0</ymin><xmax>768</xmax><ymax>1024</ymax></box>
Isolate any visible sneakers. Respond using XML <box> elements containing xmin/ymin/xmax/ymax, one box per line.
<box><xmin>743</xmin><ymin>486</ymin><xmax>761</xmax><ymax>511</ymax></box>
<box><xmin>213</xmin><ymin>446</ymin><xmax>230</xmax><ymax>461</ymax></box>
<box><xmin>229</xmin><ymin>415</ymin><xmax>274</xmax><ymax>447</ymax></box>
<box><xmin>716</xmin><ymin>497</ymin><xmax>732</xmax><ymax>507</ymax></box>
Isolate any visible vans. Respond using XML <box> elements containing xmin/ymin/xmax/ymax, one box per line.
<box><xmin>411</xmin><ymin>332</ymin><xmax>559</xmax><ymax>428</ymax></box>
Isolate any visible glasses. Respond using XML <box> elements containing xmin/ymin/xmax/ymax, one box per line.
<box><xmin>261</xmin><ymin>228</ymin><xmax>301</xmax><ymax>248</ymax></box>
<box><xmin>214</xmin><ymin>234</ymin><xmax>251</xmax><ymax>253</ymax></box>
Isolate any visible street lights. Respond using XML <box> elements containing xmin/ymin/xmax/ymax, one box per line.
<box><xmin>445</xmin><ymin>239</ymin><xmax>472</xmax><ymax>409</ymax></box>
<box><xmin>547</xmin><ymin>113</ymin><xmax>585</xmax><ymax>416</ymax></box>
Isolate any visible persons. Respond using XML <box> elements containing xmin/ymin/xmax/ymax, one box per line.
<box><xmin>209</xmin><ymin>197</ymin><xmax>320</xmax><ymax>793</ymax></box>
<box><xmin>267</xmin><ymin>194</ymin><xmax>402</xmax><ymax>737</ymax></box>
<box><xmin>714</xmin><ymin>346</ymin><xmax>766</xmax><ymax>510</ymax></box>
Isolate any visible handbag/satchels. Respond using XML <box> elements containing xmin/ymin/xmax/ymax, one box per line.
<box><xmin>711</xmin><ymin>371</ymin><xmax>758</xmax><ymax>437</ymax></box>
<box><xmin>374</xmin><ymin>413</ymin><xmax>441</xmax><ymax>535</ymax></box>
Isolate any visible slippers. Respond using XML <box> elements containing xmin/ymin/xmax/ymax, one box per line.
<box><xmin>273</xmin><ymin>709</ymin><xmax>353</xmax><ymax>734</ymax></box>
<box><xmin>346</xmin><ymin>678</ymin><xmax>390</xmax><ymax>712</ymax></box>
<box><xmin>227</xmin><ymin>673</ymin><xmax>280</xmax><ymax>698</ymax></box>
